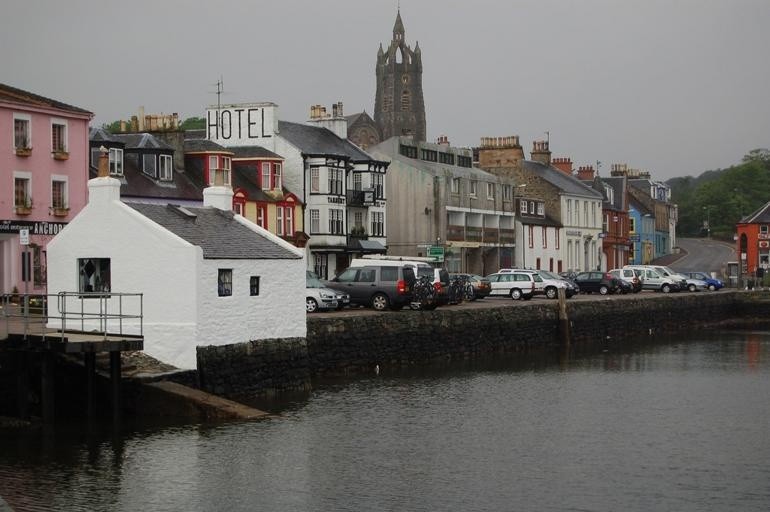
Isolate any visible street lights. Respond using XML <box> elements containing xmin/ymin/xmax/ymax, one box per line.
<box><xmin>734</xmin><ymin>185</ymin><xmax>744</xmax><ymax>221</ymax></box>
<box><xmin>702</xmin><ymin>206</ymin><xmax>711</xmax><ymax>240</ymax></box>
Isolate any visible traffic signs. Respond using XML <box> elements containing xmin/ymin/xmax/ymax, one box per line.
<box><xmin>427</xmin><ymin>247</ymin><xmax>445</xmax><ymax>262</ymax></box>
